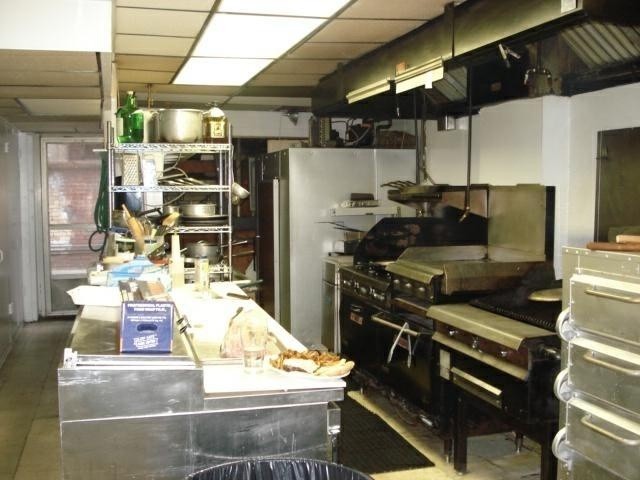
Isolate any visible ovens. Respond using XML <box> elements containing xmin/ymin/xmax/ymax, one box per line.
<box><xmin>332</xmin><ymin>279</ymin><xmax>393</xmax><ymax>368</ymax></box>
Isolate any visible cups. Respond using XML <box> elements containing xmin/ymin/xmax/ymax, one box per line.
<box><xmin>238</xmin><ymin>318</ymin><xmax>269</xmax><ymax>376</ymax></box>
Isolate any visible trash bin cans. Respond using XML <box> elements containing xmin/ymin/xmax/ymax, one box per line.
<box><xmin>327</xmin><ymin>401</ymin><xmax>341</xmax><ymax>464</ymax></box>
<box><xmin>184</xmin><ymin>457</ymin><xmax>373</xmax><ymax>480</ymax></box>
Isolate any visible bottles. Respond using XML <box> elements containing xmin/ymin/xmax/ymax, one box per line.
<box><xmin>117</xmin><ymin>90</ymin><xmax>144</xmax><ymax>143</ymax></box>
<box><xmin>202</xmin><ymin>101</ymin><xmax>228</xmax><ymax>144</ymax></box>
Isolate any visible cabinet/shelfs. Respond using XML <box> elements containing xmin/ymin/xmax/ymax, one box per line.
<box><xmin>107</xmin><ymin>120</ymin><xmax>232</xmax><ymax>282</ymax></box>
<box><xmin>41</xmin><ymin>137</ymin><xmax>107</xmax><ymax>317</ymax></box>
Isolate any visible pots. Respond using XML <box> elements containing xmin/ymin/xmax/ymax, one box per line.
<box><xmin>157</xmin><ymin>107</ymin><xmax>204</xmax><ymax>144</ymax></box>
<box><xmin>182</xmin><ymin>201</ymin><xmax>217</xmax><ymax>217</ymax></box>
<box><xmin>186</xmin><ymin>236</ymin><xmax>256</xmax><ymax>265</ymax></box>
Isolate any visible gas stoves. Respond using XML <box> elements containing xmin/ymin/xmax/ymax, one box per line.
<box><xmin>339</xmin><ymin>257</ymin><xmax>393</xmax><ymax>293</ymax></box>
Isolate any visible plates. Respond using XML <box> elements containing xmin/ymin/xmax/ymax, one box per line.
<box><xmin>264</xmin><ymin>353</ymin><xmax>351</xmax><ymax>382</ymax></box>
